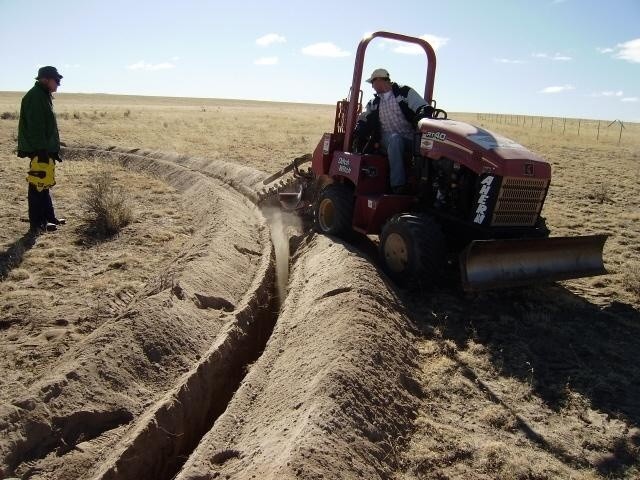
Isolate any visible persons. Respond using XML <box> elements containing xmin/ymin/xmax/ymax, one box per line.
<box><xmin>15</xmin><ymin>65</ymin><xmax>66</xmax><ymax>234</ymax></box>
<box><xmin>358</xmin><ymin>68</ymin><xmax>433</xmax><ymax>193</ymax></box>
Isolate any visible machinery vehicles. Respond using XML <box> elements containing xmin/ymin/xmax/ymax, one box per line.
<box><xmin>259</xmin><ymin>31</ymin><xmax>612</xmax><ymax>299</ymax></box>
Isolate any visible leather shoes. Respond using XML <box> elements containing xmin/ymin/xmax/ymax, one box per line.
<box><xmin>29</xmin><ymin>218</ymin><xmax>65</xmax><ymax>230</ymax></box>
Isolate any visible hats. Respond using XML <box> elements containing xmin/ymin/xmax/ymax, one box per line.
<box><xmin>33</xmin><ymin>66</ymin><xmax>63</xmax><ymax>80</ymax></box>
<box><xmin>364</xmin><ymin>69</ymin><xmax>388</xmax><ymax>82</ymax></box>
